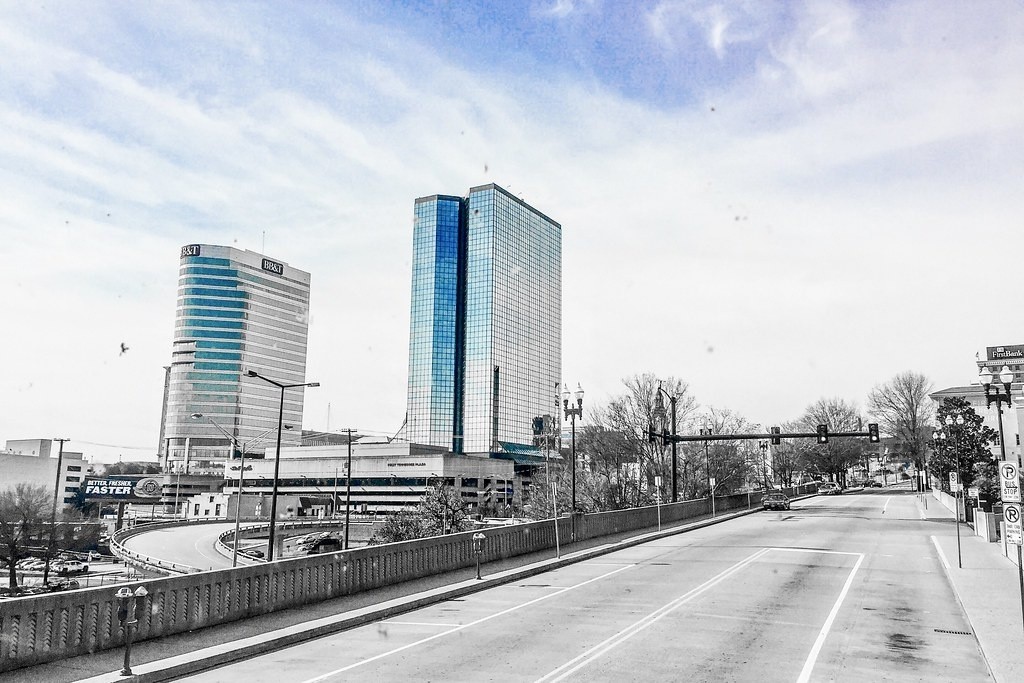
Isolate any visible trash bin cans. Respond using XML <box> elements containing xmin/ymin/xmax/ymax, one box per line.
<box><xmin>917</xmin><ymin>476</ymin><xmax>924</xmax><ymax>491</ymax></box>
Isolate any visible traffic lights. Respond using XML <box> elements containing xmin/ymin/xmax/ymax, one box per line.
<box><xmin>662</xmin><ymin>428</ymin><xmax>670</xmax><ymax>446</ymax></box>
<box><xmin>649</xmin><ymin>425</ymin><xmax>656</xmax><ymax>444</ymax></box>
<box><xmin>818</xmin><ymin>425</ymin><xmax>829</xmax><ymax>443</ymax></box>
<box><xmin>869</xmin><ymin>423</ymin><xmax>879</xmax><ymax>443</ymax></box>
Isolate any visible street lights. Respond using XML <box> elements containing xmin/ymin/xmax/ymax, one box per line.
<box><xmin>656</xmin><ymin>386</ymin><xmax>678</xmax><ymax>503</ymax></box>
<box><xmin>562</xmin><ymin>383</ymin><xmax>586</xmax><ymax>512</ymax></box>
<box><xmin>191</xmin><ymin>412</ymin><xmax>294</xmax><ymax>568</ymax></box>
<box><xmin>699</xmin><ymin>422</ymin><xmax>714</xmax><ymax>496</ymax></box>
<box><xmin>979</xmin><ymin>364</ymin><xmax>1014</xmax><ymax>462</ymax></box>
<box><xmin>759</xmin><ymin>437</ymin><xmax>769</xmax><ymax>490</ymax></box>
<box><xmin>244</xmin><ymin>369</ymin><xmax>321</xmax><ymax>563</ymax></box>
<box><xmin>157</xmin><ymin>453</ymin><xmax>197</xmax><ymax>520</ymax></box>
<box><xmin>945</xmin><ymin>414</ymin><xmax>964</xmax><ymax>499</ymax></box>
<box><xmin>933</xmin><ymin>431</ymin><xmax>947</xmax><ymax>492</ymax></box>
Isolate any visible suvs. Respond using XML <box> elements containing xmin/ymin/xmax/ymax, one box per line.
<box><xmin>825</xmin><ymin>482</ymin><xmax>844</xmax><ymax>495</ymax></box>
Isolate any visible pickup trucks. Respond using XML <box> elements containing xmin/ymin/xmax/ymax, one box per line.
<box><xmin>54</xmin><ymin>560</ymin><xmax>89</xmax><ymax>576</ymax></box>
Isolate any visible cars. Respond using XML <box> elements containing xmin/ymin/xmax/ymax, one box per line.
<box><xmin>0</xmin><ymin>579</ymin><xmax>80</xmax><ymax>597</ymax></box>
<box><xmin>763</xmin><ymin>494</ymin><xmax>791</xmax><ymax>510</ymax></box>
<box><xmin>818</xmin><ymin>485</ymin><xmax>835</xmax><ymax>495</ymax></box>
<box><xmin>862</xmin><ymin>478</ymin><xmax>882</xmax><ymax>488</ymax></box>
<box><xmin>245</xmin><ymin>550</ymin><xmax>265</xmax><ymax>558</ymax></box>
<box><xmin>6</xmin><ymin>556</ymin><xmax>62</xmax><ymax>571</ymax></box>
